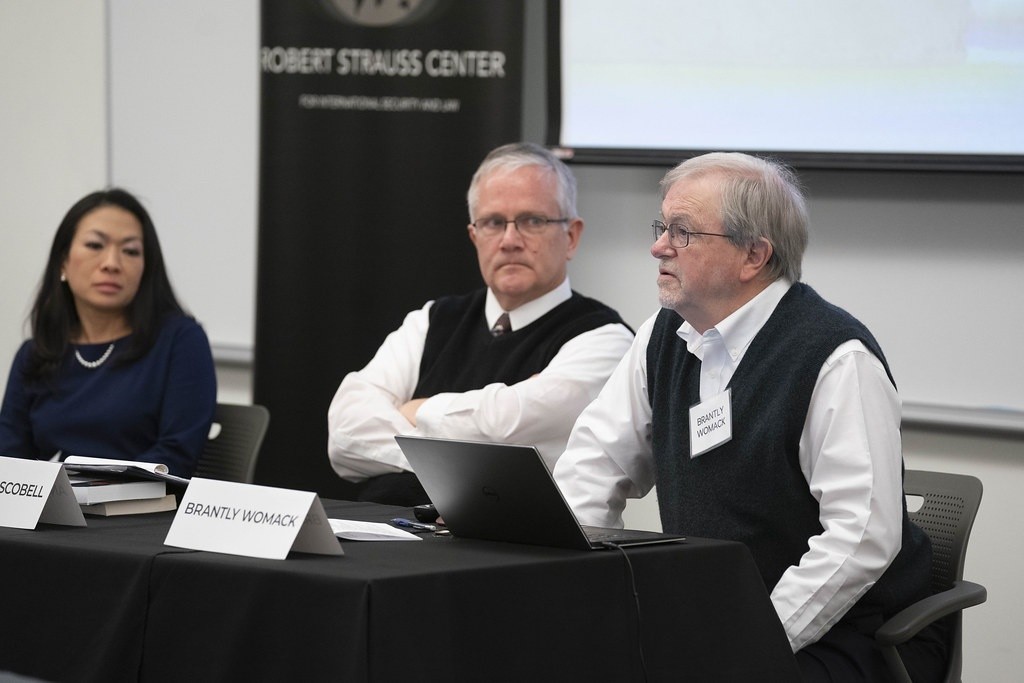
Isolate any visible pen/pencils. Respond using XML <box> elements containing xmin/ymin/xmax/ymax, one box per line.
<box><xmin>390</xmin><ymin>518</ymin><xmax>437</xmax><ymax>532</ymax></box>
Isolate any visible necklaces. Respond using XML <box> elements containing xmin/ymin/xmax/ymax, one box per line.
<box><xmin>75</xmin><ymin>345</ymin><xmax>115</xmax><ymax>367</ymax></box>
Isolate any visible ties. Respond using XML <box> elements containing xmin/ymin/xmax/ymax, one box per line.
<box><xmin>493</xmin><ymin>313</ymin><xmax>511</xmax><ymax>337</ymax></box>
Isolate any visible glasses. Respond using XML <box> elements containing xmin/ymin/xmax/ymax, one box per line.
<box><xmin>473</xmin><ymin>215</ymin><xmax>567</xmax><ymax>237</ymax></box>
<box><xmin>651</xmin><ymin>220</ymin><xmax>733</xmax><ymax>248</ymax></box>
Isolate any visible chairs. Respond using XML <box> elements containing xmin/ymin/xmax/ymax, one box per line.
<box><xmin>876</xmin><ymin>469</ymin><xmax>986</xmax><ymax>683</ymax></box>
<box><xmin>194</xmin><ymin>404</ymin><xmax>268</xmax><ymax>485</ymax></box>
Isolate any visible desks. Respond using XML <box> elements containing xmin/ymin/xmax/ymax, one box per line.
<box><xmin>0</xmin><ymin>489</ymin><xmax>752</xmax><ymax>683</ymax></box>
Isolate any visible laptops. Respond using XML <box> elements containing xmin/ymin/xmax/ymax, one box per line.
<box><xmin>392</xmin><ymin>433</ymin><xmax>686</xmax><ymax>553</ymax></box>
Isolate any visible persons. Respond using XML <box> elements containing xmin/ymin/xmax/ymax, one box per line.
<box><xmin>327</xmin><ymin>143</ymin><xmax>636</xmax><ymax>508</ymax></box>
<box><xmin>0</xmin><ymin>188</ymin><xmax>218</xmax><ymax>476</ymax></box>
<box><xmin>552</xmin><ymin>152</ymin><xmax>950</xmax><ymax>683</ymax></box>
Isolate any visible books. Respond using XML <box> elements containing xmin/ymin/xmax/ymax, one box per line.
<box><xmin>63</xmin><ymin>455</ymin><xmax>190</xmax><ymax>515</ymax></box>
